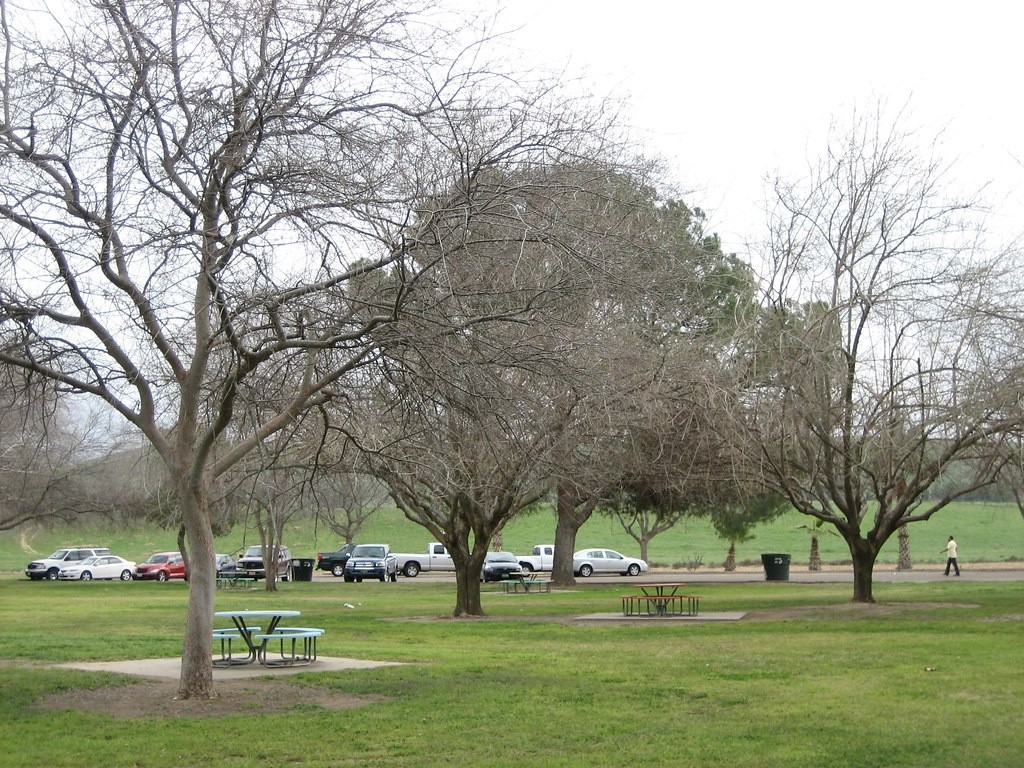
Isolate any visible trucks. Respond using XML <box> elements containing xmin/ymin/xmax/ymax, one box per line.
<box><xmin>388</xmin><ymin>541</ymin><xmax>458</xmax><ymax>577</ymax></box>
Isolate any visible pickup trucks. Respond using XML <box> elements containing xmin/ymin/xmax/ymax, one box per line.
<box><xmin>513</xmin><ymin>544</ymin><xmax>556</xmax><ymax>575</ymax></box>
<box><xmin>344</xmin><ymin>544</ymin><xmax>398</xmax><ymax>583</ymax></box>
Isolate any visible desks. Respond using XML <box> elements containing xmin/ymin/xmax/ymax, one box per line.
<box><xmin>215</xmin><ymin>610</ymin><xmax>301</xmax><ymax>664</ymax></box>
<box><xmin>220</xmin><ymin>571</ymin><xmax>247</xmax><ymax>588</ymax></box>
<box><xmin>635</xmin><ymin>583</ymin><xmax>686</xmax><ymax>616</ymax></box>
<box><xmin>510</xmin><ymin>572</ymin><xmax>545</xmax><ymax>594</ymax></box>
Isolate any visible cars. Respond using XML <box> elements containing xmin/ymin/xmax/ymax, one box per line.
<box><xmin>130</xmin><ymin>552</ymin><xmax>188</xmax><ymax>582</ymax></box>
<box><xmin>480</xmin><ymin>551</ymin><xmax>523</xmax><ymax>583</ymax></box>
<box><xmin>571</xmin><ymin>548</ymin><xmax>648</xmax><ymax>577</ymax></box>
<box><xmin>57</xmin><ymin>555</ymin><xmax>137</xmax><ymax>583</ymax></box>
<box><xmin>313</xmin><ymin>543</ymin><xmax>357</xmax><ymax>577</ymax></box>
<box><xmin>184</xmin><ymin>554</ymin><xmax>238</xmax><ymax>582</ymax></box>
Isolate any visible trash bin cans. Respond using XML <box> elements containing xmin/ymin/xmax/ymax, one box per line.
<box><xmin>760</xmin><ymin>553</ymin><xmax>791</xmax><ymax>581</ymax></box>
<box><xmin>291</xmin><ymin>558</ymin><xmax>316</xmax><ymax>582</ymax></box>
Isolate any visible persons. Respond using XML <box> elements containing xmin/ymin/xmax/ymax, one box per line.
<box><xmin>940</xmin><ymin>536</ymin><xmax>961</xmax><ymax>576</ymax></box>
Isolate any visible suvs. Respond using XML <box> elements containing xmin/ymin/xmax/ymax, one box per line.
<box><xmin>234</xmin><ymin>544</ymin><xmax>293</xmax><ymax>583</ymax></box>
<box><xmin>24</xmin><ymin>546</ymin><xmax>112</xmax><ymax>581</ymax></box>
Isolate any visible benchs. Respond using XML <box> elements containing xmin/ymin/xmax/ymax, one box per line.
<box><xmin>216</xmin><ymin>578</ymin><xmax>255</xmax><ymax>589</ymax></box>
<box><xmin>500</xmin><ymin>579</ymin><xmax>555</xmax><ymax>594</ymax></box>
<box><xmin>212</xmin><ymin>627</ymin><xmax>324</xmax><ymax>668</ymax></box>
<box><xmin>621</xmin><ymin>594</ymin><xmax>703</xmax><ymax>617</ymax></box>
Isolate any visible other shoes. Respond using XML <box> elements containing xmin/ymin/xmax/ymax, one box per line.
<box><xmin>953</xmin><ymin>574</ymin><xmax>958</xmax><ymax>576</ymax></box>
<box><xmin>942</xmin><ymin>574</ymin><xmax>948</xmax><ymax>576</ymax></box>
